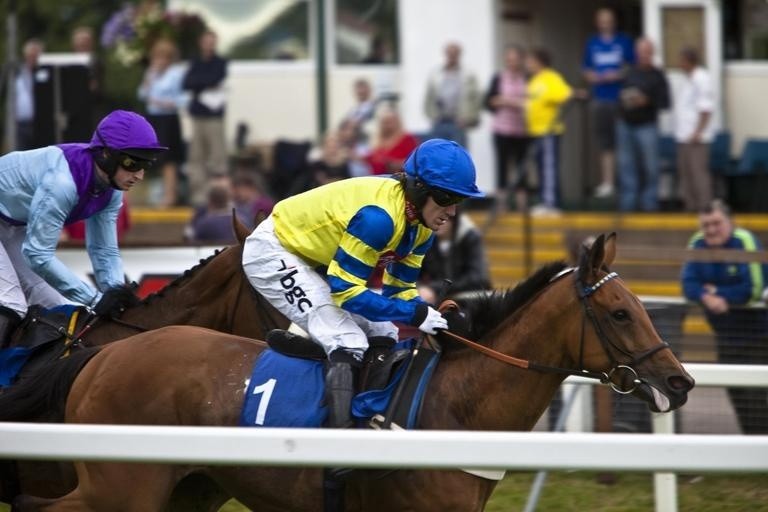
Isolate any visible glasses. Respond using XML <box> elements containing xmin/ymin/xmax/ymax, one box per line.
<box><xmin>414</xmin><ymin>146</ymin><xmax>464</xmax><ymax>207</ymax></box>
<box><xmin>94</xmin><ymin>127</ymin><xmax>158</xmax><ymax>173</ymax></box>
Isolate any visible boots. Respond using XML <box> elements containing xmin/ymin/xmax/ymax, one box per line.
<box><xmin>322</xmin><ymin>348</ymin><xmax>361</xmax><ymax>492</ymax></box>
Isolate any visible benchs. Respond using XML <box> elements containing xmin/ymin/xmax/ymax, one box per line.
<box><xmin>460</xmin><ymin>209</ymin><xmax>768</xmax><ymax>310</ymax></box>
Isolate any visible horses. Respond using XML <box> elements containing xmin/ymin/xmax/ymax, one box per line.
<box><xmin>0</xmin><ymin>207</ymin><xmax>291</xmax><ymax>511</ymax></box>
<box><xmin>0</xmin><ymin>232</ymin><xmax>696</xmax><ymax>512</ymax></box>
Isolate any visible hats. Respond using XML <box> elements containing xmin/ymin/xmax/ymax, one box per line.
<box><xmin>402</xmin><ymin>137</ymin><xmax>488</xmax><ymax>201</ymax></box>
<box><xmin>88</xmin><ymin>109</ymin><xmax>171</xmax><ymax>154</ymax></box>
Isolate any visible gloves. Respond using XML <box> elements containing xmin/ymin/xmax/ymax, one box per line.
<box><xmin>105</xmin><ymin>284</ymin><xmax>141</xmax><ymax>306</ymax></box>
<box><xmin>92</xmin><ymin>292</ymin><xmax>127</xmax><ymax>322</ymax></box>
<box><xmin>416</xmin><ymin>304</ymin><xmax>449</xmax><ymax>335</ymax></box>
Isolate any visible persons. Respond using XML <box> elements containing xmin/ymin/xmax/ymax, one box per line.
<box><xmin>1</xmin><ymin>109</ymin><xmax>170</xmax><ymax>351</ymax></box>
<box><xmin>1</xmin><ymin>0</ymin><xmax>767</xmax><ymax>248</ymax></box>
<box><xmin>241</xmin><ymin>137</ymin><xmax>488</xmax><ymax>482</ymax></box>
<box><xmin>416</xmin><ymin>210</ymin><xmax>490</xmax><ymax>306</ymax></box>
<box><xmin>681</xmin><ymin>199</ymin><xmax>768</xmax><ymax>434</ymax></box>
